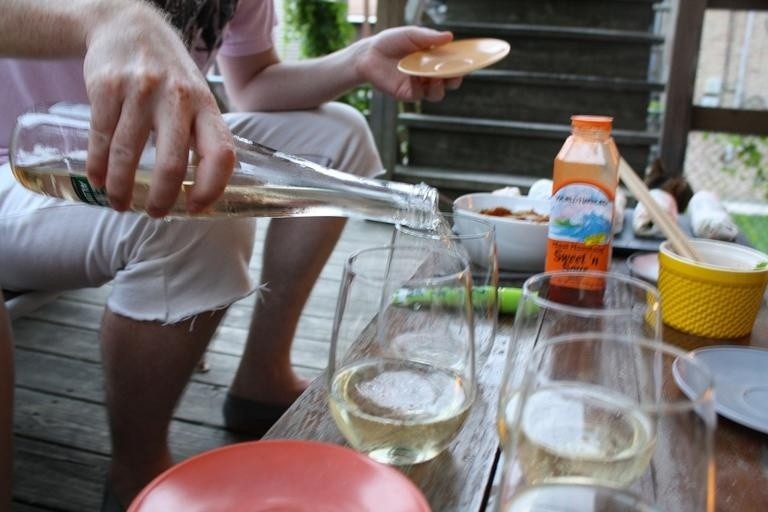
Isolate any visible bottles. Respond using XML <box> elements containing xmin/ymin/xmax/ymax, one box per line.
<box><xmin>545</xmin><ymin>114</ymin><xmax>620</xmax><ymax>291</ymax></box>
<box><xmin>7</xmin><ymin>100</ymin><xmax>439</xmax><ymax>231</ymax></box>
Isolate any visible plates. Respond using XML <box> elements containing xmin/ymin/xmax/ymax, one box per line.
<box><xmin>673</xmin><ymin>342</ymin><xmax>767</xmax><ymax>437</ymax></box>
<box><xmin>396</xmin><ymin>33</ymin><xmax>511</xmax><ymax>78</ymax></box>
<box><xmin>613</xmin><ymin>205</ymin><xmax>736</xmax><ymax>251</ymax></box>
<box><xmin>126</xmin><ymin>437</ymin><xmax>430</xmax><ymax>512</ymax></box>
<box><xmin>628</xmin><ymin>253</ymin><xmax>659</xmax><ymax>284</ymax></box>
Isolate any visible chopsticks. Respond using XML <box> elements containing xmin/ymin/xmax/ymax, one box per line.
<box><xmin>617</xmin><ymin>155</ymin><xmax>705</xmax><ymax>262</ymax></box>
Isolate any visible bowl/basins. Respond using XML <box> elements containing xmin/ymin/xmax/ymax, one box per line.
<box><xmin>452</xmin><ymin>189</ymin><xmax>550</xmax><ymax>279</ymax></box>
<box><xmin>657</xmin><ymin>236</ymin><xmax>768</xmax><ymax>341</ymax></box>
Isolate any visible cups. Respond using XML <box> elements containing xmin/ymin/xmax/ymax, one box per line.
<box><xmin>378</xmin><ymin>207</ymin><xmax>499</xmax><ymax>382</ymax></box>
<box><xmin>324</xmin><ymin>242</ymin><xmax>477</xmax><ymax>468</ymax></box>
<box><xmin>497</xmin><ymin>480</ymin><xmax>665</xmax><ymax>512</ymax></box>
<box><xmin>505</xmin><ymin>332</ymin><xmax>717</xmax><ymax>512</ymax></box>
<box><xmin>498</xmin><ymin>271</ymin><xmax>664</xmax><ymax>466</ymax></box>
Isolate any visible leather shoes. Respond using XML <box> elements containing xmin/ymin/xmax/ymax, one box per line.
<box><xmin>222</xmin><ymin>391</ymin><xmax>297</xmax><ymax>445</ymax></box>
<box><xmin>99</xmin><ymin>482</ymin><xmax>128</xmax><ymax>512</ymax></box>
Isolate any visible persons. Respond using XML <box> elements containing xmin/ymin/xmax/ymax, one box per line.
<box><xmin>0</xmin><ymin>0</ymin><xmax>466</xmax><ymax>512</ymax></box>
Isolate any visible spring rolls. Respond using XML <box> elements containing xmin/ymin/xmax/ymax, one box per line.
<box><xmin>631</xmin><ymin>188</ymin><xmax>678</xmax><ymax>238</ymax></box>
<box><xmin>686</xmin><ymin>189</ymin><xmax>739</xmax><ymax>242</ymax></box>
<box><xmin>611</xmin><ymin>186</ymin><xmax>627</xmax><ymax>235</ymax></box>
<box><xmin>528</xmin><ymin>179</ymin><xmax>554</xmax><ymax>217</ymax></box>
